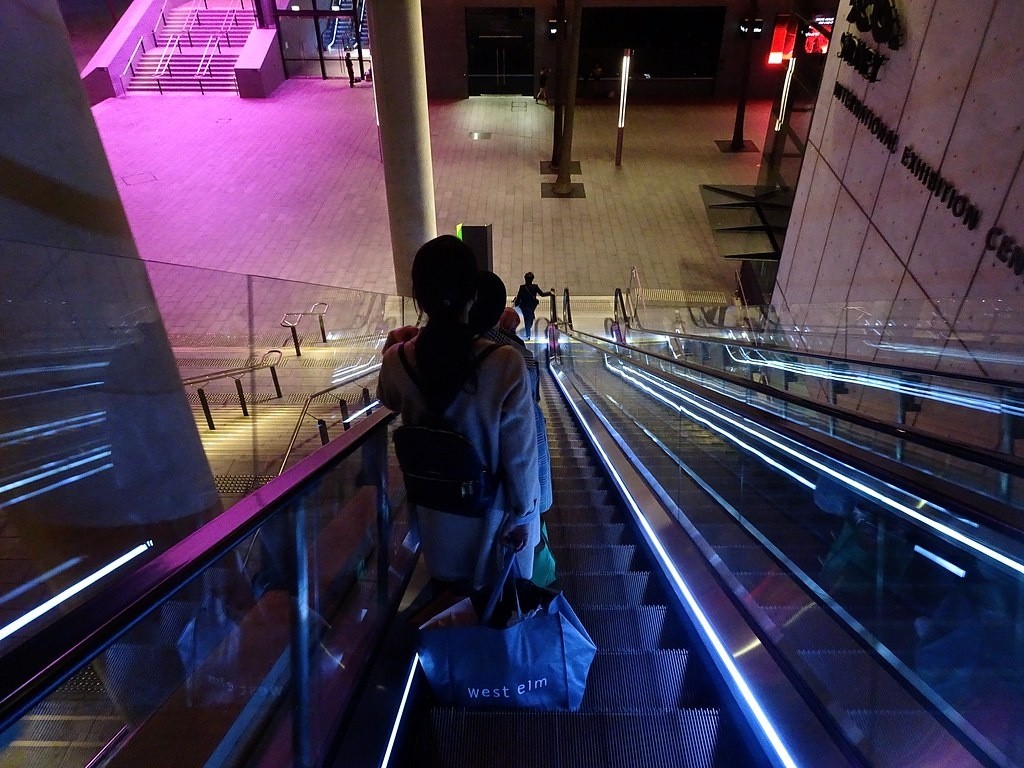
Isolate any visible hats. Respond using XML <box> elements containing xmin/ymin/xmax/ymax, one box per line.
<box><xmin>499</xmin><ymin>307</ymin><xmax>520</xmax><ymax>330</ymax></box>
<box><xmin>464</xmin><ymin>270</ymin><xmax>507</xmax><ymax>334</ymax></box>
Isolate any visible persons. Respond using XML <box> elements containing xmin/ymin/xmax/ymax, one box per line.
<box><xmin>514</xmin><ymin>272</ymin><xmax>551</xmax><ymax>340</ymax></box>
<box><xmin>345</xmin><ymin>53</ymin><xmax>355</xmax><ymax>89</ymax></box>
<box><xmin>375</xmin><ymin>235</ymin><xmax>558</xmax><ymax>600</ymax></box>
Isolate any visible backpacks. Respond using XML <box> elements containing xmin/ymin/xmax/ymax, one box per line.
<box><xmin>392</xmin><ymin>344</ymin><xmax>504</xmax><ymax>516</ymax></box>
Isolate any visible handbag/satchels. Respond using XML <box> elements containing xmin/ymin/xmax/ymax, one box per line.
<box><xmin>526</xmin><ymin>298</ymin><xmax>539</xmax><ymax>307</ymax></box>
<box><xmin>413</xmin><ymin>548</ymin><xmax>598</xmax><ymax>713</ymax></box>
